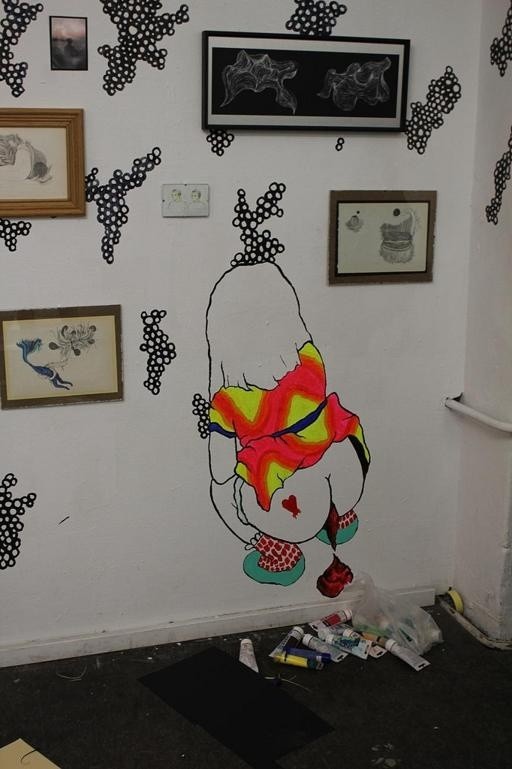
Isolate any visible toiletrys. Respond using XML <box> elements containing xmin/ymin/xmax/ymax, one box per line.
<box><xmin>238</xmin><ymin>607</ymin><xmax>443</xmax><ymax>675</ymax></box>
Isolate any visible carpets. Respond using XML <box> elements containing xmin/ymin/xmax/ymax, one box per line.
<box><xmin>138</xmin><ymin>643</ymin><xmax>339</xmax><ymax>768</ymax></box>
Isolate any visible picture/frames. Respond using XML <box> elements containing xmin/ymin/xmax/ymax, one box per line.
<box><xmin>0</xmin><ymin>107</ymin><xmax>87</xmax><ymax>220</ymax></box>
<box><xmin>0</xmin><ymin>302</ymin><xmax>126</xmax><ymax>412</ymax></box>
<box><xmin>47</xmin><ymin>13</ymin><xmax>90</xmax><ymax>73</ymax></box>
<box><xmin>328</xmin><ymin>190</ymin><xmax>437</xmax><ymax>284</ymax></box>
<box><xmin>199</xmin><ymin>28</ymin><xmax>411</xmax><ymax>133</ymax></box>
